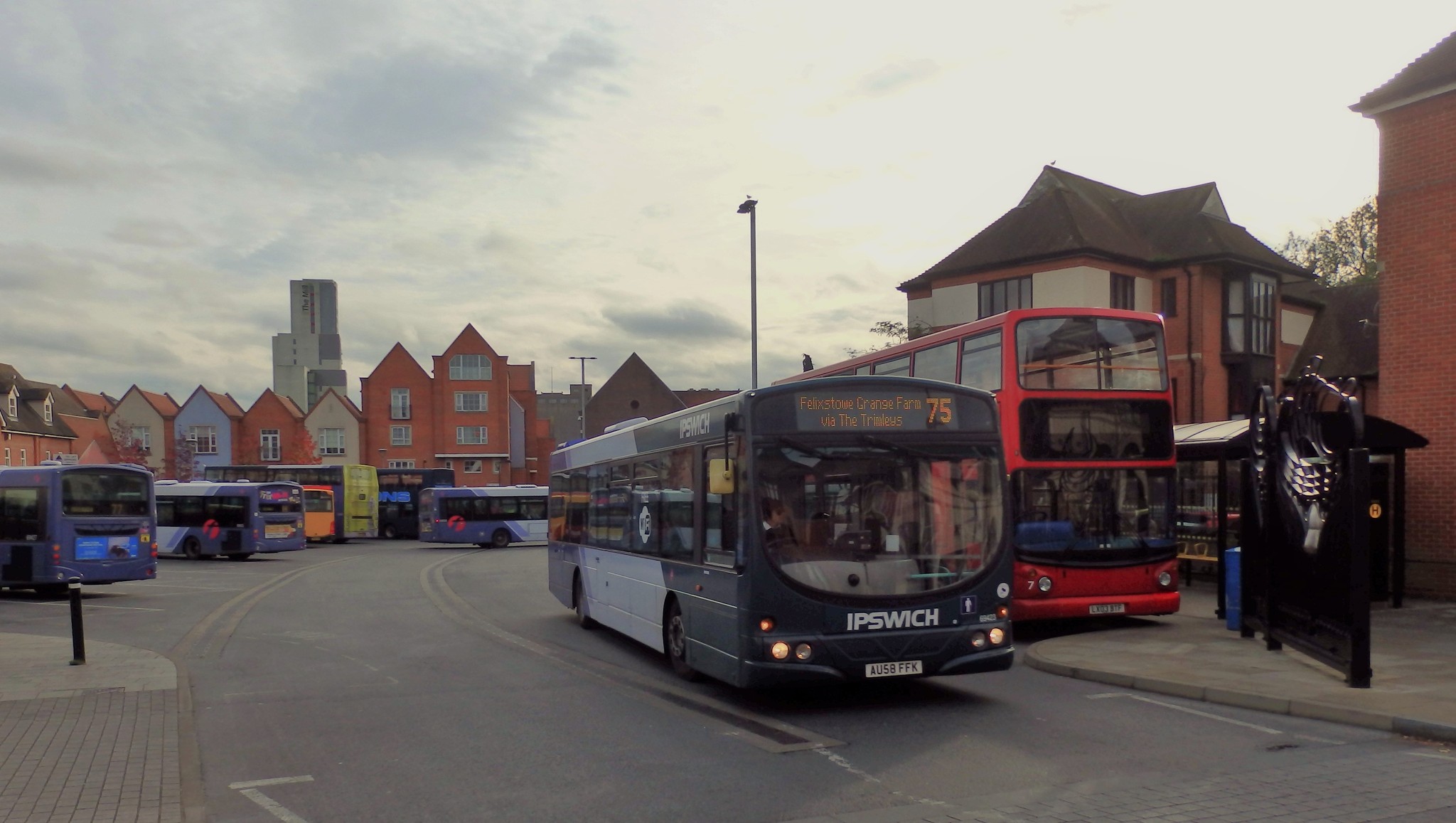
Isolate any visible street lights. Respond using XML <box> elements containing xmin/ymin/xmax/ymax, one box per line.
<box><xmin>737</xmin><ymin>193</ymin><xmax>759</xmax><ymax>390</ymax></box>
<box><xmin>568</xmin><ymin>357</ymin><xmax>598</xmax><ymax>439</ymax></box>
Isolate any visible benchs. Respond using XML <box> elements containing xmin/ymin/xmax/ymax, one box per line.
<box><xmin>451</xmin><ymin>514</ymin><xmax>523</xmax><ymax>520</ymax></box>
<box><xmin>1177</xmin><ymin>541</ymin><xmax>1218</xmax><ymax>586</ymax></box>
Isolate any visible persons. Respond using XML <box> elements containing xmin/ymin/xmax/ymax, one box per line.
<box><xmin>761</xmin><ymin>497</ymin><xmax>797</xmax><ymax>548</ymax></box>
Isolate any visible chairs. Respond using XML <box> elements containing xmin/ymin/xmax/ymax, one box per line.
<box><xmin>566</xmin><ymin>503</ymin><xmax>887</xmax><ymax>551</ymax></box>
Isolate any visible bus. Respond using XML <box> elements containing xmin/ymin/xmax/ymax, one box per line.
<box><xmin>770</xmin><ymin>307</ymin><xmax>1180</xmax><ymax>621</ymax></box>
<box><xmin>548</xmin><ymin>375</ymin><xmax>1015</xmax><ymax>688</ymax></box>
<box><xmin>549</xmin><ymin>492</ymin><xmax>591</xmax><ymax>542</ymax></box>
<box><xmin>203</xmin><ymin>464</ymin><xmax>379</xmax><ymax>544</ymax></box>
<box><xmin>418</xmin><ymin>484</ymin><xmax>549</xmax><ymax>548</ymax></box>
<box><xmin>0</xmin><ymin>460</ymin><xmax>158</xmax><ymax>597</ymax></box>
<box><xmin>117</xmin><ymin>479</ymin><xmax>307</xmax><ymax>560</ymax></box>
<box><xmin>376</xmin><ymin>467</ymin><xmax>455</xmax><ymax>539</ymax></box>
<box><xmin>261</xmin><ymin>485</ymin><xmax>335</xmax><ymax>544</ymax></box>
<box><xmin>588</xmin><ymin>485</ymin><xmax>722</xmax><ymax>553</ymax></box>
